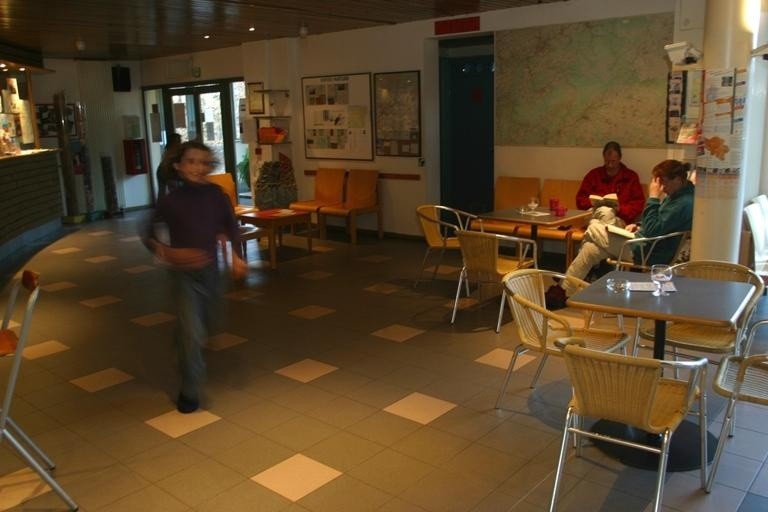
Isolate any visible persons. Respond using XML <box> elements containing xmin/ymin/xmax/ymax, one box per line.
<box><xmin>140</xmin><ymin>141</ymin><xmax>247</xmax><ymax>414</ymax></box>
<box><xmin>575</xmin><ymin>142</ymin><xmax>646</xmax><ymax>226</ymax></box>
<box><xmin>544</xmin><ymin>159</ymin><xmax>694</xmax><ymax>312</ymax></box>
<box><xmin>158</xmin><ymin>134</ymin><xmax>184</xmax><ymax>198</ymax></box>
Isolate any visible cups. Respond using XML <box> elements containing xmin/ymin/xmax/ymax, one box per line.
<box><xmin>553</xmin><ymin>206</ymin><xmax>571</xmax><ymax>217</ymax></box>
<box><xmin>527</xmin><ymin>197</ymin><xmax>542</xmax><ymax>216</ymax></box>
<box><xmin>548</xmin><ymin>197</ymin><xmax>561</xmax><ymax>212</ymax></box>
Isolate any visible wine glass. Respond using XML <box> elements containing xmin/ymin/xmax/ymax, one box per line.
<box><xmin>650</xmin><ymin>263</ymin><xmax>674</xmax><ymax>299</ymax></box>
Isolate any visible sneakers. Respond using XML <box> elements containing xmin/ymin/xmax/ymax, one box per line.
<box><xmin>544</xmin><ymin>292</ymin><xmax>567</xmax><ymax>310</ymax></box>
<box><xmin>175</xmin><ymin>390</ymin><xmax>200</xmax><ymax>414</ymax></box>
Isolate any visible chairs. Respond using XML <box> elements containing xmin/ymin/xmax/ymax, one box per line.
<box><xmin>740</xmin><ymin>202</ymin><xmax>768</xmax><ymax>296</ymax></box>
<box><xmin>569</xmin><ymin>180</ymin><xmax>647</xmax><ymax>268</ymax></box>
<box><xmin>602</xmin><ymin>230</ymin><xmax>691</xmax><ymax>318</ymax></box>
<box><xmin>549</xmin><ymin>336</ymin><xmax>708</xmax><ymax>511</ymax></box>
<box><xmin>199</xmin><ymin>173</ymin><xmax>260</xmax><ymax>264</ymax></box>
<box><xmin>450</xmin><ymin>230</ymin><xmax>537</xmax><ymax>334</ymax></box>
<box><xmin>318</xmin><ymin>168</ymin><xmax>384</xmax><ymax>244</ymax></box>
<box><xmin>514</xmin><ymin>180</ymin><xmax>582</xmax><ymax>265</ymax></box>
<box><xmin>632</xmin><ymin>259</ymin><xmax>765</xmax><ymax>378</ymax></box>
<box><xmin>0</xmin><ymin>269</ymin><xmax>80</xmax><ymax>510</ymax></box>
<box><xmin>704</xmin><ymin>318</ymin><xmax>767</xmax><ymax>494</ymax></box>
<box><xmin>494</xmin><ymin>267</ymin><xmax>632</xmax><ymax>409</ymax></box>
<box><xmin>749</xmin><ymin>194</ymin><xmax>768</xmax><ymax>236</ymax></box>
<box><xmin>469</xmin><ymin>176</ymin><xmax>540</xmax><ymax>274</ymax></box>
<box><xmin>290</xmin><ymin>169</ymin><xmax>345</xmax><ymax>234</ymax></box>
<box><xmin>413</xmin><ymin>204</ymin><xmax>478</xmax><ymax>290</ymax></box>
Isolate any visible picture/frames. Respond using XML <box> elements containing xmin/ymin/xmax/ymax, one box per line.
<box><xmin>665</xmin><ymin>70</ymin><xmax>688</xmax><ymax>144</ymax></box>
<box><xmin>373</xmin><ymin>70</ymin><xmax>422</xmax><ymax>158</ymax></box>
<box><xmin>247</xmin><ymin>82</ymin><xmax>264</xmax><ymax>115</ymax></box>
<box><xmin>300</xmin><ymin>72</ymin><xmax>374</xmax><ymax>161</ymax></box>
<box><xmin>34</xmin><ymin>103</ymin><xmax>77</xmax><ymax>138</ymax></box>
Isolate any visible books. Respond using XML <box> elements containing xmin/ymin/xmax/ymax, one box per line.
<box><xmin>605</xmin><ymin>224</ymin><xmax>635</xmax><ymax>239</ymax></box>
<box><xmin>588</xmin><ymin>193</ymin><xmax>618</xmax><ymax>208</ymax></box>
